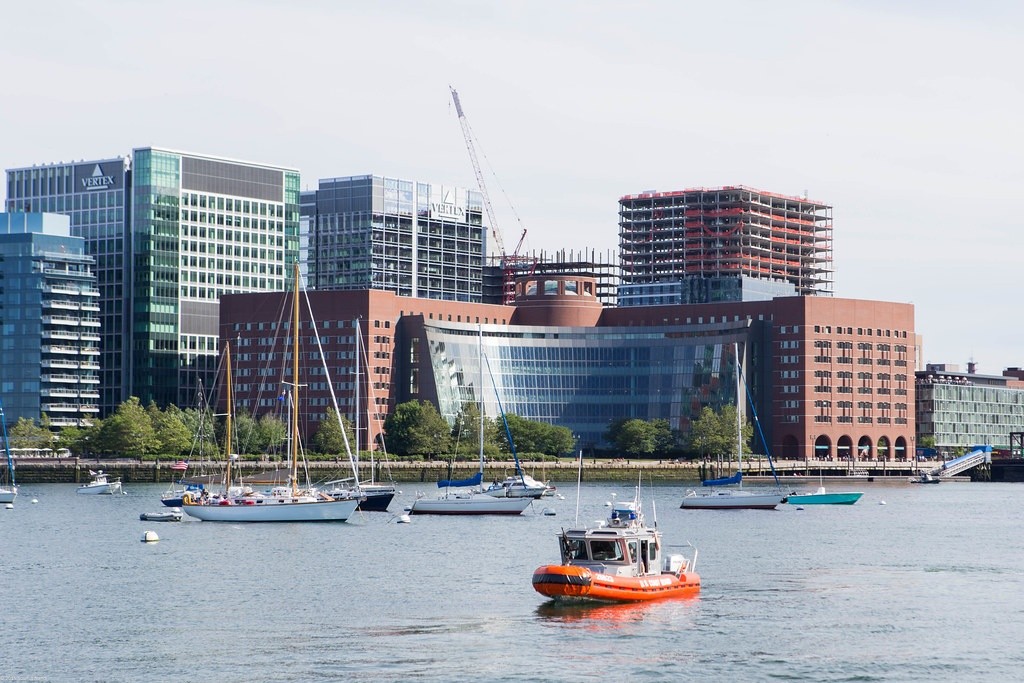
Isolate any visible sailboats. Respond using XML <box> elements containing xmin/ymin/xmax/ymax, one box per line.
<box><xmin>181</xmin><ymin>262</ymin><xmax>367</xmax><ymax>522</ymax></box>
<box><xmin>161</xmin><ymin>379</ymin><xmax>226</xmax><ymax>507</ymax></box>
<box><xmin>680</xmin><ymin>341</ymin><xmax>788</xmax><ymax>510</ymax></box>
<box><xmin>407</xmin><ymin>325</ymin><xmax>535</xmax><ymax>515</ymax></box>
<box><xmin>0</xmin><ymin>400</ymin><xmax>18</xmax><ymax>503</ymax></box>
<box><xmin>324</xmin><ymin>318</ymin><xmax>395</xmax><ymax>512</ymax></box>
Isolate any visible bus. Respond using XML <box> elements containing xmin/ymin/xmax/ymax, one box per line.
<box><xmin>941</xmin><ymin>452</ymin><xmax>958</xmax><ymax>460</ymax></box>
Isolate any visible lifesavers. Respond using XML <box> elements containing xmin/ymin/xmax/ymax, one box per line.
<box><xmin>182</xmin><ymin>493</ymin><xmax>191</xmax><ymax>504</ymax></box>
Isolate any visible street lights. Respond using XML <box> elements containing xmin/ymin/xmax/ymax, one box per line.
<box><xmin>910</xmin><ymin>436</ymin><xmax>915</xmax><ymax>461</ymax></box>
<box><xmin>810</xmin><ymin>435</ymin><xmax>816</xmax><ymax>460</ymax></box>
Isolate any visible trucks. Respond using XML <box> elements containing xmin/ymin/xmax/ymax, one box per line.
<box><xmin>916</xmin><ymin>448</ymin><xmax>938</xmax><ymax>457</ymax></box>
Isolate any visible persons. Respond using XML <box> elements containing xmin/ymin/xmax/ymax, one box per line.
<box><xmin>620</xmin><ymin>543</ymin><xmax>645</xmax><ymax>573</ymax></box>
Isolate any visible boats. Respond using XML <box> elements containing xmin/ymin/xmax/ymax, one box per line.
<box><xmin>910</xmin><ymin>472</ymin><xmax>941</xmax><ymax>484</ymax></box>
<box><xmin>76</xmin><ymin>470</ymin><xmax>122</xmax><ymax>494</ymax></box>
<box><xmin>482</xmin><ymin>485</ymin><xmax>507</xmax><ymax>497</ymax></box>
<box><xmin>787</xmin><ymin>467</ymin><xmax>864</xmax><ymax>505</ymax></box>
<box><xmin>542</xmin><ymin>480</ymin><xmax>557</xmax><ymax>496</ymax></box>
<box><xmin>532</xmin><ymin>451</ymin><xmax>700</xmax><ymax>604</ymax></box>
<box><xmin>501</xmin><ymin>467</ymin><xmax>547</xmax><ymax>499</ymax></box>
<box><xmin>139</xmin><ymin>508</ymin><xmax>183</xmax><ymax>522</ymax></box>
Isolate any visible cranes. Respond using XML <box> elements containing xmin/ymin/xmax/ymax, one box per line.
<box><xmin>449</xmin><ymin>86</ymin><xmax>540</xmax><ymax>305</ymax></box>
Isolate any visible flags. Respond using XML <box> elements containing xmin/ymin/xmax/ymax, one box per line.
<box><xmin>170</xmin><ymin>460</ymin><xmax>188</xmax><ymax>469</ymax></box>
